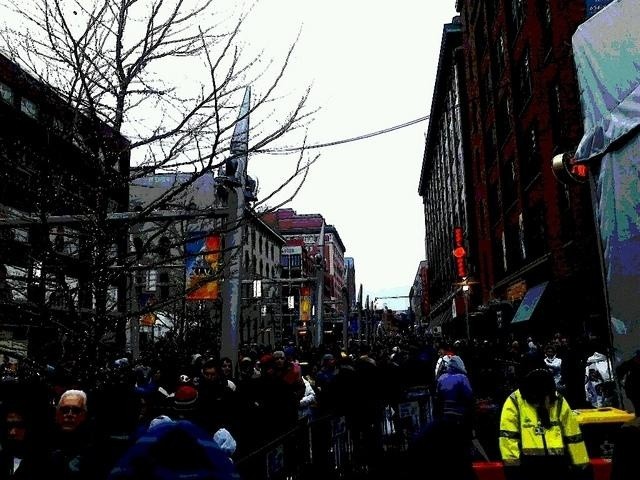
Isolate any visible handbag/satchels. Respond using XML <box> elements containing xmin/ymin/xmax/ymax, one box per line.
<box><xmin>437</xmin><ymin>357</ymin><xmax>448</xmax><ymax>375</ymax></box>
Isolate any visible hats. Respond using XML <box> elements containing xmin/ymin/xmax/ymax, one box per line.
<box><xmin>257</xmin><ymin>355</ymin><xmax>275</xmax><ymax>367</ymax></box>
<box><xmin>448</xmin><ymin>358</ymin><xmax>462</xmax><ymax>369</ymax></box>
<box><xmin>174</xmin><ymin>386</ymin><xmax>201</xmax><ymax>412</ymax></box>
<box><xmin>323</xmin><ymin>354</ymin><xmax>334</xmax><ymax>359</ymax></box>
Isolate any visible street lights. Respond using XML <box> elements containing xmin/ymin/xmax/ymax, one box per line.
<box><xmin>454</xmin><ymin>277</ymin><xmax>479</xmax><ymax>341</ymax></box>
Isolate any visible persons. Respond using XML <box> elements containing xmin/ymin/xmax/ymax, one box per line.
<box><xmin>1</xmin><ymin>334</ymin><xmax>639</xmax><ymax>479</ymax></box>
